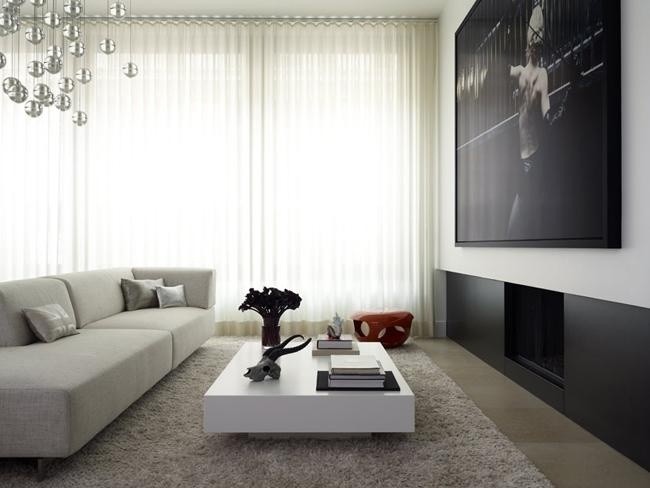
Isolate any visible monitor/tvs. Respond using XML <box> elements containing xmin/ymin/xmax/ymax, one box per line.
<box><xmin>454</xmin><ymin>0</ymin><xmax>621</xmax><ymax>248</ymax></box>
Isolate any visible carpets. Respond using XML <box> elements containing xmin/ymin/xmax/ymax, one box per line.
<box><xmin>0</xmin><ymin>337</ymin><xmax>556</xmax><ymax>488</ymax></box>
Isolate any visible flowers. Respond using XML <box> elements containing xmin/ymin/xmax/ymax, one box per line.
<box><xmin>238</xmin><ymin>287</ymin><xmax>302</xmax><ymax>346</ymax></box>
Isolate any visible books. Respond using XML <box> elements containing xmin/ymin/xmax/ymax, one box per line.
<box><xmin>316</xmin><ymin>354</ymin><xmax>400</xmax><ymax>392</ymax></box>
<box><xmin>311</xmin><ymin>337</ymin><xmax>360</xmax><ymax>356</ymax></box>
<box><xmin>316</xmin><ymin>334</ymin><xmax>353</xmax><ymax>349</ymax></box>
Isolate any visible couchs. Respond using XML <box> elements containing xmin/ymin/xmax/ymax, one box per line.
<box><xmin>0</xmin><ymin>267</ymin><xmax>216</xmax><ymax>482</ymax></box>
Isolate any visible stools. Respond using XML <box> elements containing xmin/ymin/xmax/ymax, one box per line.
<box><xmin>351</xmin><ymin>311</ymin><xmax>414</xmax><ymax>348</ymax></box>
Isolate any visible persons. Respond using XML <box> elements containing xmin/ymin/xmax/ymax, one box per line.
<box><xmin>506</xmin><ymin>6</ymin><xmax>588</xmax><ymax>242</ymax></box>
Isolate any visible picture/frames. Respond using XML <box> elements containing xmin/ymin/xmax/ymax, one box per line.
<box><xmin>261</xmin><ymin>325</ymin><xmax>281</xmax><ymax>350</ymax></box>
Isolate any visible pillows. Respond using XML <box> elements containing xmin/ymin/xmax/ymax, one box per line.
<box><xmin>121</xmin><ymin>278</ymin><xmax>187</xmax><ymax>311</ymax></box>
<box><xmin>21</xmin><ymin>303</ymin><xmax>80</xmax><ymax>342</ymax></box>
